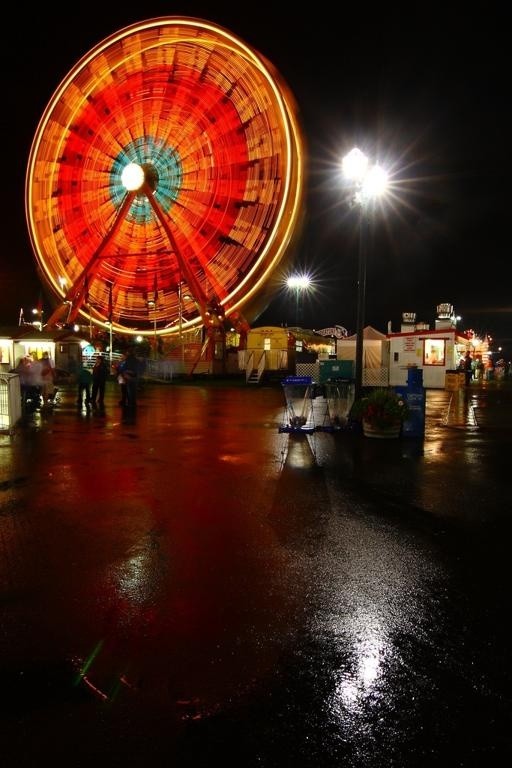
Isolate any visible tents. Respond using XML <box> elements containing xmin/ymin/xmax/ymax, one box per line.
<box><xmin>335</xmin><ymin>324</ymin><xmax>390</xmax><ymax>388</ymax></box>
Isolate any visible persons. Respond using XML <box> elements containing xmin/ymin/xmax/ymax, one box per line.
<box><xmin>473</xmin><ymin>356</ymin><xmax>484</xmax><ymax>380</ymax></box>
<box><xmin>114</xmin><ymin>348</ymin><xmax>145</xmax><ymax>425</ymax></box>
<box><xmin>16</xmin><ymin>355</ymin><xmax>46</xmax><ymax>403</ymax></box>
<box><xmin>458</xmin><ymin>358</ymin><xmax>465</xmax><ymax>369</ymax></box>
<box><xmin>88</xmin><ymin>355</ymin><xmax>108</xmax><ymax>402</ymax></box>
<box><xmin>76</xmin><ymin>366</ymin><xmax>93</xmax><ymax>403</ymax></box>
<box><xmin>464</xmin><ymin>349</ymin><xmax>472</xmax><ymax>384</ymax></box>
<box><xmin>111</xmin><ymin>351</ymin><xmax>130</xmax><ymax>405</ymax></box>
<box><xmin>487</xmin><ymin>354</ymin><xmax>495</xmax><ymax>381</ymax></box>
<box><xmin>38</xmin><ymin>351</ymin><xmax>57</xmax><ymax>404</ymax></box>
<box><xmin>8</xmin><ymin>357</ymin><xmax>35</xmax><ymax>418</ymax></box>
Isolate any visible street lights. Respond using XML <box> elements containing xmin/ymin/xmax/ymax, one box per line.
<box><xmin>286</xmin><ymin>275</ymin><xmax>312</xmax><ymax>325</ymax></box>
<box><xmin>343</xmin><ymin>145</ymin><xmax>390</xmax><ymax>403</ymax></box>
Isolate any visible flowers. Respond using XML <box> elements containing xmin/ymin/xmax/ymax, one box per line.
<box><xmin>351</xmin><ymin>385</ymin><xmax>413</xmax><ymax>434</ymax></box>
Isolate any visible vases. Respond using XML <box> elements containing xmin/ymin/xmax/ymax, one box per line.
<box><xmin>362</xmin><ymin>417</ymin><xmax>401</xmax><ymax>438</ymax></box>
